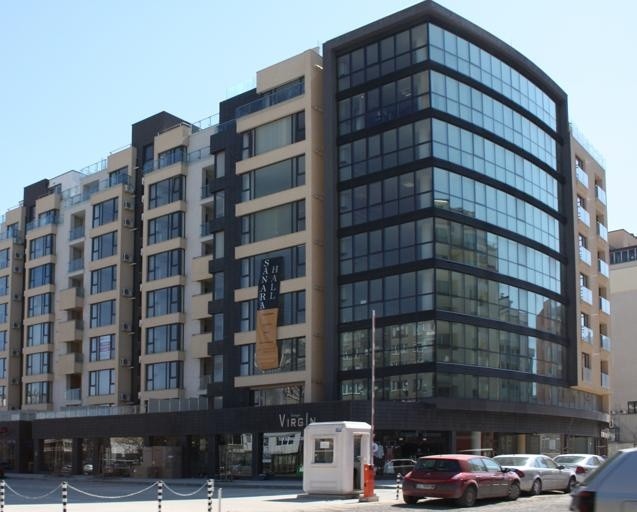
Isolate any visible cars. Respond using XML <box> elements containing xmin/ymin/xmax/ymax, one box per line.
<box><xmin>400</xmin><ymin>455</ymin><xmax>521</xmax><ymax>502</ymax></box>
<box><xmin>571</xmin><ymin>447</ymin><xmax>637</xmax><ymax>511</ymax></box>
<box><xmin>376</xmin><ymin>458</ymin><xmax>416</xmax><ymax>478</ymax></box>
<box><xmin>551</xmin><ymin>454</ymin><xmax>606</xmax><ymax>486</ymax></box>
<box><xmin>489</xmin><ymin>453</ymin><xmax>576</xmax><ymax>494</ymax></box>
<box><xmin>51</xmin><ymin>458</ymin><xmax>136</xmax><ymax>476</ymax></box>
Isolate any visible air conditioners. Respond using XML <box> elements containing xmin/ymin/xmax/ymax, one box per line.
<box><xmin>119</xmin><ymin>183</ymin><xmax>134</xmax><ymax>403</ymax></box>
<box><xmin>9</xmin><ymin>236</ymin><xmax>23</xmax><ymax>410</ymax></box>
